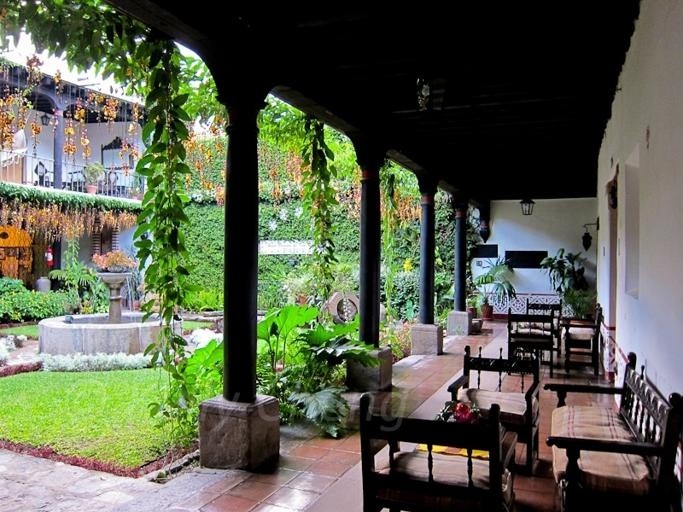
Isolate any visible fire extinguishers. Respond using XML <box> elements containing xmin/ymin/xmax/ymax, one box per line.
<box><xmin>46</xmin><ymin>244</ymin><xmax>53</xmax><ymax>266</ymax></box>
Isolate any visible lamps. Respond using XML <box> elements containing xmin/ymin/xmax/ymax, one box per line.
<box><xmin>39</xmin><ymin>111</ymin><xmax>48</xmax><ymax>126</ymax></box>
<box><xmin>579</xmin><ymin>218</ymin><xmax>600</xmax><ymax>252</ymax></box>
<box><xmin>517</xmin><ymin>193</ymin><xmax>536</xmax><ymax>216</ymax></box>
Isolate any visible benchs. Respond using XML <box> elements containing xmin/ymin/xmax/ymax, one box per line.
<box><xmin>537</xmin><ymin>351</ymin><xmax>682</xmax><ymax>508</ymax></box>
<box><xmin>557</xmin><ymin>300</ymin><xmax>605</xmax><ymax>379</ymax></box>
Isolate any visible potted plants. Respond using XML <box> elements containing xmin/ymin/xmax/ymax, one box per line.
<box><xmin>472</xmin><ymin>256</ymin><xmax>517</xmax><ymax>323</ymax></box>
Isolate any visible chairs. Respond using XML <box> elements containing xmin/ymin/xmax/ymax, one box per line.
<box><xmin>32</xmin><ymin>159</ymin><xmax>120</xmax><ymax>197</ymax></box>
<box><xmin>352</xmin><ymin>391</ymin><xmax>516</xmax><ymax>512</ymax></box>
<box><xmin>525</xmin><ymin>298</ymin><xmax>567</xmax><ymax>360</ymax></box>
<box><xmin>505</xmin><ymin>307</ymin><xmax>556</xmax><ymax>381</ymax></box>
<box><xmin>444</xmin><ymin>345</ymin><xmax>546</xmax><ymax>478</ymax></box>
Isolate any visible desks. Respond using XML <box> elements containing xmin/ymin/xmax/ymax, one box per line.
<box><xmin>410</xmin><ymin>413</ymin><xmax>519</xmax><ymax>490</ymax></box>
